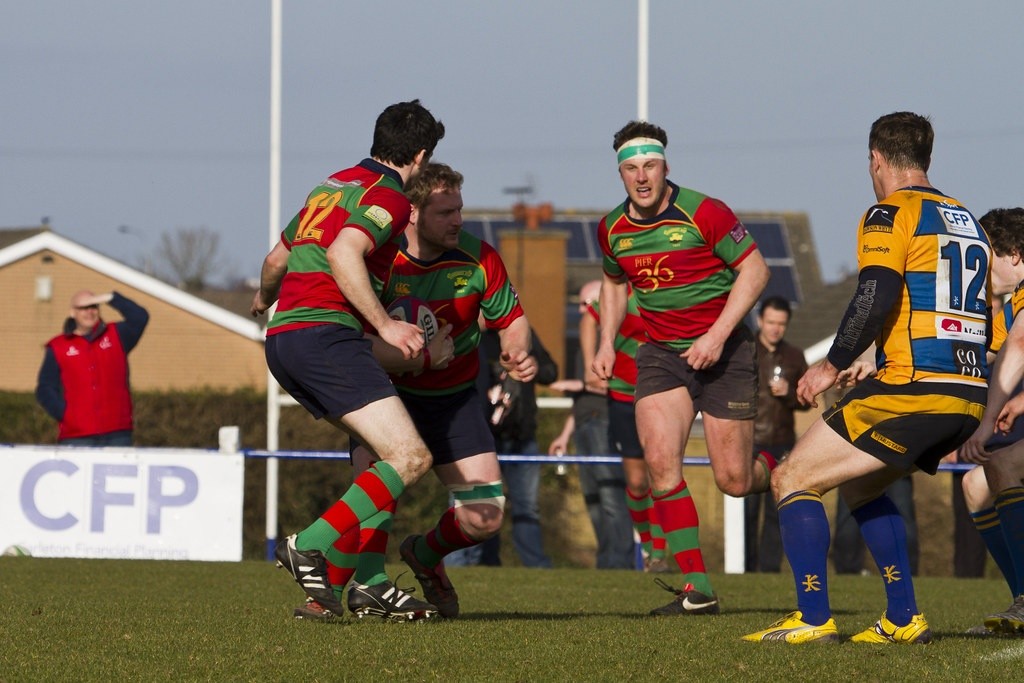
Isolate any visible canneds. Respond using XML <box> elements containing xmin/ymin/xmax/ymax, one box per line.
<box><xmin>553</xmin><ymin>449</ymin><xmax>569</xmax><ymax>474</ymax></box>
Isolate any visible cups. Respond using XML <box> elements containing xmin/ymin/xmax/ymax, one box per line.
<box><xmin>771</xmin><ymin>366</ymin><xmax>783</xmax><ymax>382</ymax></box>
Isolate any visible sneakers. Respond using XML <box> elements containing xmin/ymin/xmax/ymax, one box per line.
<box><xmin>965</xmin><ymin>593</ymin><xmax>1024</xmax><ymax>638</ymax></box>
<box><xmin>399</xmin><ymin>534</ymin><xmax>460</xmax><ymax>620</ymax></box>
<box><xmin>740</xmin><ymin>610</ymin><xmax>839</xmax><ymax>645</ymax></box>
<box><xmin>272</xmin><ymin>532</ymin><xmax>344</xmax><ymax>618</ymax></box>
<box><xmin>649</xmin><ymin>577</ymin><xmax>721</xmax><ymax>617</ymax></box>
<box><xmin>292</xmin><ymin>595</ymin><xmax>336</xmax><ymax>619</ymax></box>
<box><xmin>846</xmin><ymin>609</ymin><xmax>931</xmax><ymax>644</ymax></box>
<box><xmin>347</xmin><ymin>570</ymin><xmax>439</xmax><ymax>625</ymax></box>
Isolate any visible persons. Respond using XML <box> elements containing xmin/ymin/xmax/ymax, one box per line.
<box><xmin>36</xmin><ymin>291</ymin><xmax>149</xmax><ymax>448</ymax></box>
<box><xmin>740</xmin><ymin>111</ymin><xmax>1024</xmax><ymax>645</ymax></box>
<box><xmin>449</xmin><ymin>309</ymin><xmax>558</xmax><ymax>568</ymax></box>
<box><xmin>592</xmin><ymin>120</ymin><xmax>777</xmax><ymax>616</ymax></box>
<box><xmin>252</xmin><ymin>102</ymin><xmax>537</xmax><ymax>624</ymax></box>
<box><xmin>548</xmin><ymin>279</ymin><xmax>637</xmax><ymax>570</ymax></box>
<box><xmin>744</xmin><ymin>293</ymin><xmax>810</xmax><ymax>575</ymax></box>
<box><xmin>580</xmin><ymin>283</ymin><xmax>669</xmax><ymax>573</ymax></box>
